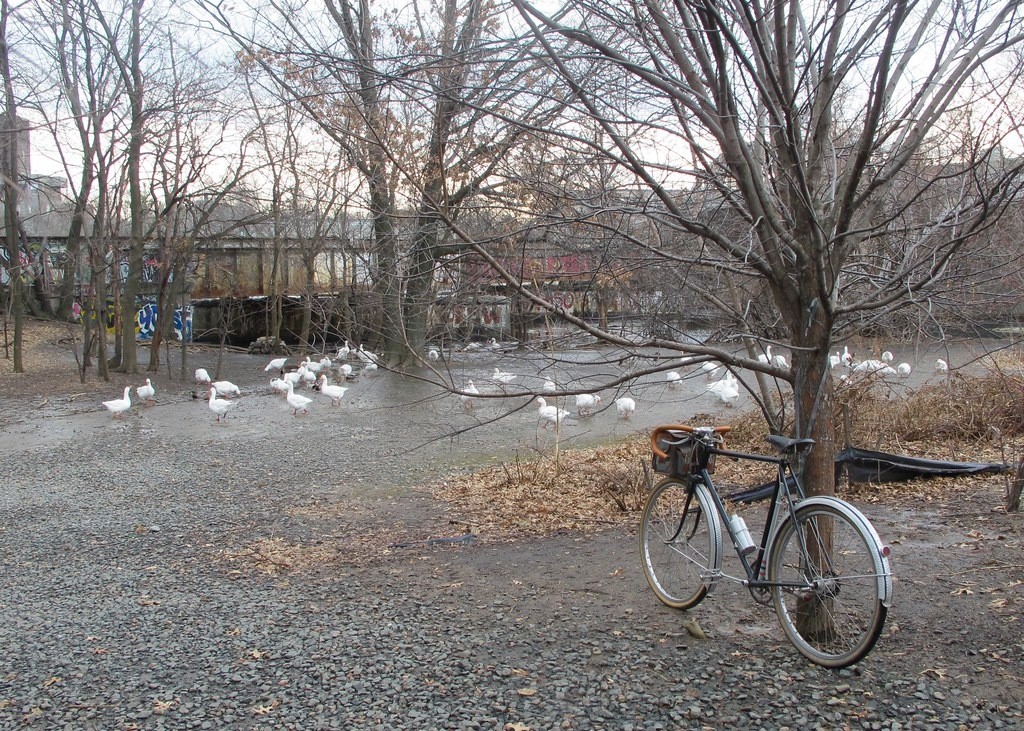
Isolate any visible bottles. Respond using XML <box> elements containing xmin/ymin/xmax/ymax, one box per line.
<box><xmin>729</xmin><ymin>510</ymin><xmax>756</xmax><ymax>556</ymax></box>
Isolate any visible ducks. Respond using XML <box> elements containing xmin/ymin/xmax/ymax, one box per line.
<box><xmin>614</xmin><ymin>397</ymin><xmax>635</xmax><ymax>420</ymax></box>
<box><xmin>534</xmin><ymin>397</ymin><xmax>571</xmax><ymax>432</ymax></box>
<box><xmin>575</xmin><ymin>393</ymin><xmax>602</xmax><ymax>416</ymax></box>
<box><xmin>542</xmin><ymin>376</ymin><xmax>557</xmax><ymax>401</ymax></box>
<box><xmin>934</xmin><ymin>359</ymin><xmax>948</xmax><ymax>375</ymax></box>
<box><xmin>319</xmin><ymin>375</ymin><xmax>348</xmax><ymax>406</ymax></box>
<box><xmin>101</xmin><ymin>385</ymin><xmax>132</xmax><ymax>420</ymax></box>
<box><xmin>680</xmin><ymin>351</ymin><xmax>692</xmax><ymax>368</ymax></box>
<box><xmin>758</xmin><ymin>345</ymin><xmax>772</xmax><ymax>364</ymax></box>
<box><xmin>461</xmin><ymin>337</ymin><xmax>501</xmax><ymax>352</ymax></box>
<box><xmin>702</xmin><ymin>360</ymin><xmax>720</xmax><ymax>380</ymax></box>
<box><xmin>428</xmin><ymin>350</ymin><xmax>439</xmax><ymax>362</ymax></box>
<box><xmin>773</xmin><ymin>355</ymin><xmax>789</xmax><ymax>370</ymax></box>
<box><xmin>707</xmin><ymin>368</ymin><xmax>739</xmax><ymax>408</ymax></box>
<box><xmin>136</xmin><ymin>378</ymin><xmax>155</xmax><ymax>403</ymax></box>
<box><xmin>458</xmin><ymin>379</ymin><xmax>479</xmax><ymax>411</ymax></box>
<box><xmin>829</xmin><ymin>345</ymin><xmax>912</xmax><ymax>387</ymax></box>
<box><xmin>263</xmin><ymin>358</ymin><xmax>288</xmax><ymax>374</ymax></box>
<box><xmin>492</xmin><ymin>368</ymin><xmax>517</xmax><ymax>389</ymax></box>
<box><xmin>208</xmin><ymin>387</ymin><xmax>234</xmax><ymax>422</ymax></box>
<box><xmin>666</xmin><ymin>372</ymin><xmax>684</xmax><ymax>391</ymax></box>
<box><xmin>194</xmin><ymin>368</ymin><xmax>211</xmax><ymax>387</ymax></box>
<box><xmin>269</xmin><ymin>340</ymin><xmax>379</xmax><ymax>399</ymax></box>
<box><xmin>284</xmin><ymin>380</ymin><xmax>313</xmax><ymax>416</ymax></box>
<box><xmin>211</xmin><ymin>381</ymin><xmax>241</xmax><ymax>399</ymax></box>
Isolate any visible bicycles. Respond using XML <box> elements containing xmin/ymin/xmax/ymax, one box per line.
<box><xmin>636</xmin><ymin>424</ymin><xmax>895</xmax><ymax>668</ymax></box>
